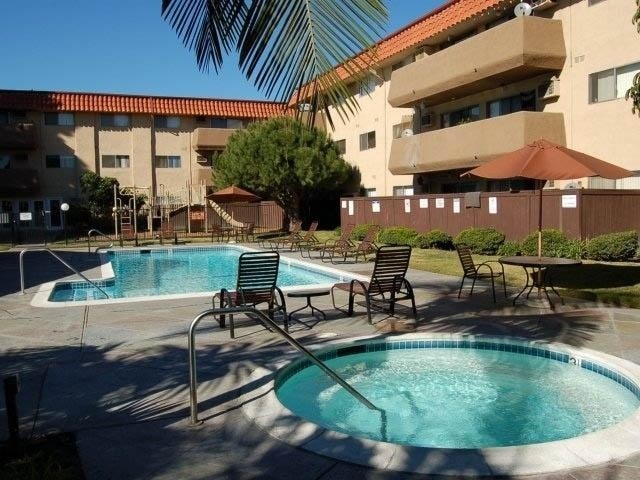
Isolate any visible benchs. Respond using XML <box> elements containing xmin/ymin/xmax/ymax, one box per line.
<box><xmin>332</xmin><ymin>245</ymin><xmax>418</xmax><ymax>325</ymax></box>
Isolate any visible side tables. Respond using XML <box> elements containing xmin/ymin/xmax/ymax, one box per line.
<box><xmin>285</xmin><ymin>291</ymin><xmax>331</xmax><ymax>321</ymax></box>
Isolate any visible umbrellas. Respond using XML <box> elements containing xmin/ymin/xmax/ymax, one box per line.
<box><xmin>204</xmin><ymin>184</ymin><xmax>263</xmax><ymax>236</ymax></box>
<box><xmin>459</xmin><ymin>135</ymin><xmax>638</xmax><ymax>297</ymax></box>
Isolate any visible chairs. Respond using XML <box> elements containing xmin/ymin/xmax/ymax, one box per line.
<box><xmin>159</xmin><ymin>220</ymin><xmax>178</xmax><ymax>245</ymax></box>
<box><xmin>213</xmin><ymin>249</ymin><xmax>288</xmax><ymax>339</ymax></box>
<box><xmin>456</xmin><ymin>243</ymin><xmax>508</xmax><ymax>304</ymax></box>
<box><xmin>120</xmin><ymin>222</ymin><xmax>139</xmax><ymax>246</ymax></box>
<box><xmin>211</xmin><ymin>221</ymin><xmax>255</xmax><ymax>243</ymax></box>
<box><xmin>268</xmin><ymin>219</ymin><xmax>381</xmax><ymax>263</ymax></box>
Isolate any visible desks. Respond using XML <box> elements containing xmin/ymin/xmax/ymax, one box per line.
<box><xmin>499</xmin><ymin>255</ymin><xmax>583</xmax><ymax>310</ymax></box>
